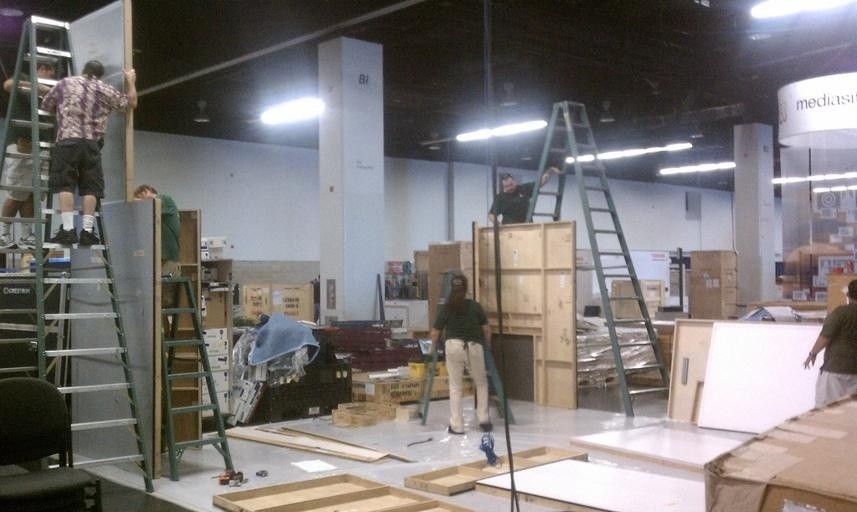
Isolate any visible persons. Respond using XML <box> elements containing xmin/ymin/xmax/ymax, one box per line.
<box><xmin>0</xmin><ymin>60</ymin><xmax>53</xmax><ymax>251</ymax></box>
<box><xmin>39</xmin><ymin>59</ymin><xmax>138</xmax><ymax>245</ymax></box>
<box><xmin>488</xmin><ymin>165</ymin><xmax>565</xmax><ymax>225</ymax></box>
<box><xmin>428</xmin><ymin>273</ymin><xmax>493</xmax><ymax>434</ymax></box>
<box><xmin>132</xmin><ymin>185</ymin><xmax>182</xmax><ymax>279</ymax></box>
<box><xmin>803</xmin><ymin>278</ymin><xmax>856</xmax><ymax>410</ymax></box>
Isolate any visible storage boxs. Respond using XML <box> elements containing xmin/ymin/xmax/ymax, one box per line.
<box><xmin>414</xmin><ymin>248</ymin><xmax>429</xmax><ymax>272</ymax></box>
<box><xmin>352</xmin><ymin>367</ymin><xmax>472</xmax><ymax>404</ymax></box>
<box><xmin>239</xmin><ymin>282</ymin><xmax>313</xmax><ymax>324</ymax></box>
<box><xmin>424</xmin><ymin>242</ymin><xmax>475</xmax><ymax>330</ymax></box>
<box><xmin>609</xmin><ymin>249</ymin><xmax>855</xmax><ymax>324</ymax></box>
<box><xmin>331</xmin><ymin>400</ymin><xmax>398</xmax><ymax>427</ymax></box>
<box><xmin>701</xmin><ymin>394</ymin><xmax>856</xmax><ymax>512</ymax></box>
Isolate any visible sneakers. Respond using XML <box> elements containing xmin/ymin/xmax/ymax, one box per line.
<box><xmin>0</xmin><ymin>234</ymin><xmax>17</xmax><ymax>249</ymax></box>
<box><xmin>79</xmin><ymin>227</ymin><xmax>99</xmax><ymax>245</ymax></box>
<box><xmin>449</xmin><ymin>426</ymin><xmax>465</xmax><ymax>434</ymax></box>
<box><xmin>19</xmin><ymin>232</ymin><xmax>35</xmax><ymax>245</ymax></box>
<box><xmin>50</xmin><ymin>224</ymin><xmax>78</xmax><ymax>243</ymax></box>
<box><xmin>480</xmin><ymin>422</ymin><xmax>493</xmax><ymax>431</ymax></box>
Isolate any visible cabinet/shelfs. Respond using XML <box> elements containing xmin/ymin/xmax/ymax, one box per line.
<box><xmin>160</xmin><ymin>209</ymin><xmax>203</xmax><ymax>452</ymax></box>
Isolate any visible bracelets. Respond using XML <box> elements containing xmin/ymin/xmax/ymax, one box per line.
<box><xmin>808</xmin><ymin>352</ymin><xmax>816</xmax><ymax>357</ymax></box>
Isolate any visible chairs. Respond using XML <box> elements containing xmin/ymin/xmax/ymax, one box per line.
<box><xmin>0</xmin><ymin>376</ymin><xmax>103</xmax><ymax>511</ymax></box>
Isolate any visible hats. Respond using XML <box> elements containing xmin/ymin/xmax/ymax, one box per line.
<box><xmin>451</xmin><ymin>274</ymin><xmax>467</xmax><ymax>286</ymax></box>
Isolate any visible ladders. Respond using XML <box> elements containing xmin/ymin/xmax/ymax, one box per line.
<box><xmin>161</xmin><ymin>274</ymin><xmax>234</xmax><ymax>481</ymax></box>
<box><xmin>417</xmin><ymin>268</ymin><xmax>516</xmax><ymax>426</ymax></box>
<box><xmin>524</xmin><ymin>101</ymin><xmax>668</xmax><ymax>418</ymax></box>
<box><xmin>0</xmin><ymin>15</ymin><xmax>153</xmax><ymax>493</ymax></box>
